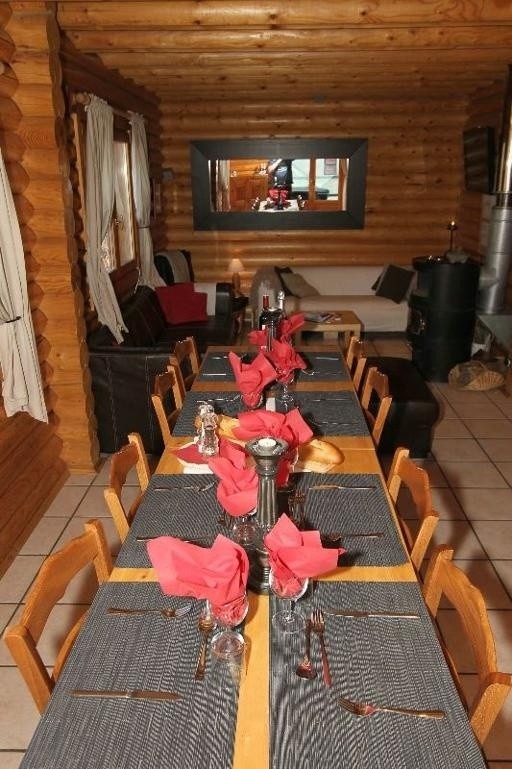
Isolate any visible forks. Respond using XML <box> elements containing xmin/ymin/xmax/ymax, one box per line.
<box><xmin>301</xmin><ymin>368</ymin><xmax>344</xmax><ymax>376</ymax></box>
<box><xmin>321</xmin><ymin>531</ymin><xmax>383</xmax><ymax>543</ymax></box>
<box><xmin>310</xmin><ymin>607</ymin><xmax>332</xmax><ymax>688</ymax></box>
<box><xmin>111</xmin><ymin>604</ymin><xmax>192</xmax><ymax>618</ymax></box>
<box><xmin>339</xmin><ymin>696</ymin><xmax>445</xmax><ymax>721</ymax></box>
<box><xmin>150</xmin><ymin>482</ymin><xmax>217</xmax><ymax>494</ymax></box>
<box><xmin>310</xmin><ymin>419</ymin><xmax>357</xmax><ymax>428</ymax></box>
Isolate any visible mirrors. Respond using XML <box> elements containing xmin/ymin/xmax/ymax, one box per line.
<box><xmin>79</xmin><ymin>120</ymin><xmax>137</xmax><ymax>282</ymax></box>
<box><xmin>193</xmin><ymin>138</ymin><xmax>367</xmax><ymax>230</ymax></box>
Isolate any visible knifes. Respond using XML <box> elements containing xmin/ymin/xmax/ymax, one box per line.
<box><xmin>69</xmin><ymin>688</ymin><xmax>180</xmax><ymax>702</ymax></box>
<box><xmin>136</xmin><ymin>535</ymin><xmax>207</xmax><ymax>545</ymax></box>
<box><xmin>327</xmin><ymin>610</ymin><xmax>422</xmax><ymax>620</ymax></box>
<box><xmin>312</xmin><ymin>483</ymin><xmax>377</xmax><ymax>492</ymax></box>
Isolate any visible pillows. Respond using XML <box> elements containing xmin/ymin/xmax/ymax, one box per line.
<box><xmin>156</xmin><ymin>284</ymin><xmax>194</xmax><ymax>323</ymax></box>
<box><xmin>275</xmin><ymin>266</ymin><xmax>293</xmax><ymax>296</ymax></box>
<box><xmin>169</xmin><ymin>291</ymin><xmax>207</xmax><ymax>324</ymax></box>
<box><xmin>376</xmin><ymin>264</ymin><xmax>416</xmax><ymax>304</ymax></box>
<box><xmin>281</xmin><ymin>273</ymin><xmax>320</xmax><ymax>298</ymax></box>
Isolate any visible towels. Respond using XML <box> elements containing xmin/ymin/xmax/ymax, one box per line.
<box><xmin>170</xmin><ymin>438</ymin><xmax>258</xmax><ymax>516</ymax></box>
<box><xmin>263</xmin><ymin>514</ymin><xmax>346</xmax><ymax>596</ymax></box>
<box><xmin>145</xmin><ymin>535</ymin><xmax>250</xmax><ymax>624</ymax></box>
<box><xmin>280</xmin><ymin>314</ymin><xmax>305</xmax><ymax>342</ymax></box>
<box><xmin>249</xmin><ymin>331</ymin><xmax>269</xmax><ymax>352</ymax></box>
<box><xmin>268</xmin><ymin>340</ymin><xmax>307</xmax><ymax>382</ymax></box>
<box><xmin>233</xmin><ymin>409</ymin><xmax>315</xmax><ymax>484</ymax></box>
<box><xmin>228</xmin><ymin>352</ymin><xmax>278</xmax><ymax>407</ymax></box>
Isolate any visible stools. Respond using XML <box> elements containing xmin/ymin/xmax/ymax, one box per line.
<box><xmin>350</xmin><ymin>354</ymin><xmax>441</xmax><ymax>458</ymax></box>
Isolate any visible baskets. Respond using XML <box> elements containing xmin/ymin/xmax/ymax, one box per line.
<box><xmin>448</xmin><ymin>360</ymin><xmax>504</xmax><ymax>391</ymax></box>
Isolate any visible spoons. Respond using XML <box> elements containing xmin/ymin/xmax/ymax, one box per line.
<box><xmin>297</xmin><ymin>614</ymin><xmax>317</xmax><ymax>680</ymax></box>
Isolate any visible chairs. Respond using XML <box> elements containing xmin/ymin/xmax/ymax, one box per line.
<box><xmin>387</xmin><ymin>447</ymin><xmax>440</xmax><ymax>574</ymax></box>
<box><xmin>360</xmin><ymin>367</ymin><xmax>396</xmax><ymax>442</ymax></box>
<box><xmin>104</xmin><ymin>433</ymin><xmax>150</xmax><ymax>542</ymax></box>
<box><xmin>6</xmin><ymin>518</ymin><xmax>112</xmax><ymax>713</ymax></box>
<box><xmin>171</xmin><ymin>336</ymin><xmax>200</xmax><ymax>396</ymax></box>
<box><xmin>151</xmin><ymin>365</ymin><xmax>184</xmax><ymax>447</ymax></box>
<box><xmin>346</xmin><ymin>337</ymin><xmax>367</xmax><ymax>391</ymax></box>
<box><xmin>422</xmin><ymin>546</ymin><xmax>511</xmax><ymax>744</ymax></box>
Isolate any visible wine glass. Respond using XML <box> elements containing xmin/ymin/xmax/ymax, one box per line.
<box><xmin>195</xmin><ymin>367</ymin><xmax>308</xmax><ymax>660</ymax></box>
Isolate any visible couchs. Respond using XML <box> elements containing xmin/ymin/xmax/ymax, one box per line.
<box><xmin>259</xmin><ymin>267</ymin><xmax>418</xmax><ymax>333</ymax></box>
<box><xmin>87</xmin><ymin>284</ymin><xmax>236</xmax><ymax>452</ymax></box>
<box><xmin>152</xmin><ymin>248</ymin><xmax>248</xmax><ymax>311</ymax></box>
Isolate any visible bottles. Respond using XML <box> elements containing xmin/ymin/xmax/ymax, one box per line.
<box><xmin>259</xmin><ymin>290</ymin><xmax>287</xmax><ymax>352</ymax></box>
<box><xmin>277</xmin><ymin>189</ymin><xmax>283</xmax><ymax>210</ymax></box>
